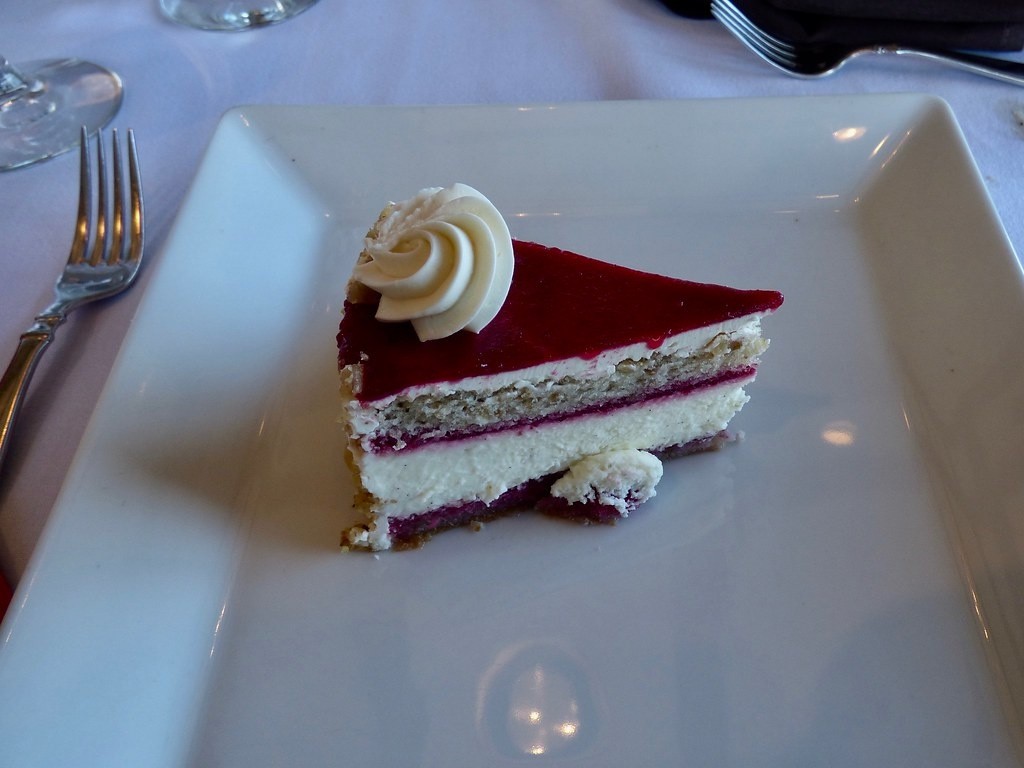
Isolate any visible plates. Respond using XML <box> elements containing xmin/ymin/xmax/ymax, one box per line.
<box><xmin>0</xmin><ymin>81</ymin><xmax>1022</xmax><ymax>768</ymax></box>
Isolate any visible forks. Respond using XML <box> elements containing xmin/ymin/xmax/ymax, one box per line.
<box><xmin>710</xmin><ymin>0</ymin><xmax>1024</xmax><ymax>87</ymax></box>
<box><xmin>0</xmin><ymin>123</ymin><xmax>145</xmax><ymax>458</ymax></box>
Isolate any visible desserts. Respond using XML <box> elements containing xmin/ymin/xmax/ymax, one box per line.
<box><xmin>334</xmin><ymin>180</ymin><xmax>786</xmax><ymax>553</ymax></box>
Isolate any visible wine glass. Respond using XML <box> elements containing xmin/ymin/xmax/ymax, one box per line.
<box><xmin>0</xmin><ymin>45</ymin><xmax>124</xmax><ymax>170</ymax></box>
